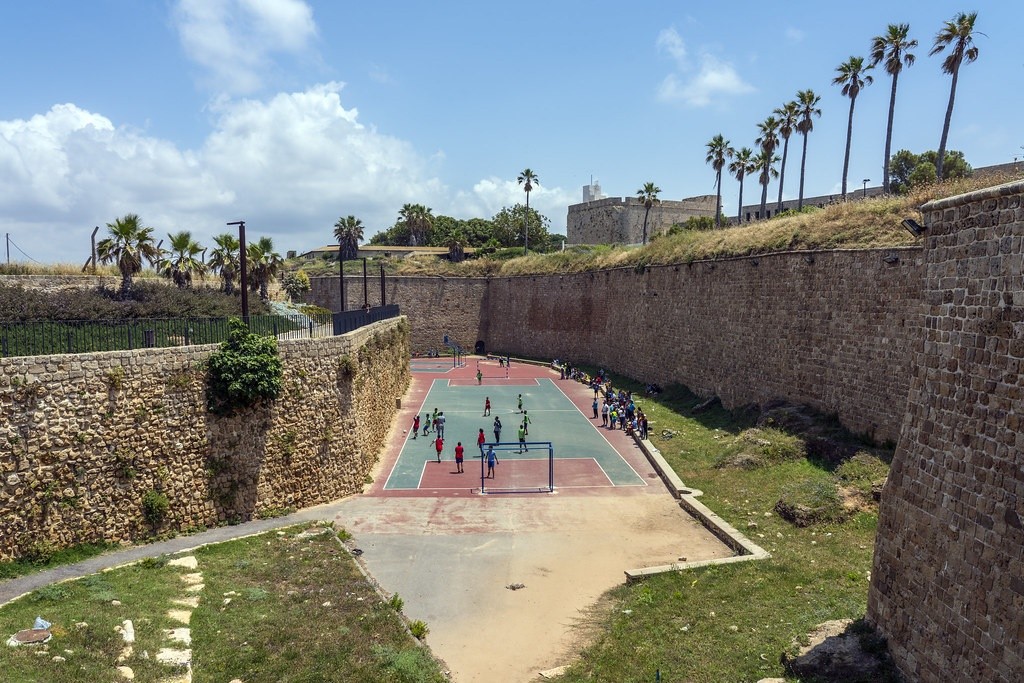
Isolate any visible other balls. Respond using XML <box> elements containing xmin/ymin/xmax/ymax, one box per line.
<box><xmin>403</xmin><ymin>430</ymin><xmax>407</xmax><ymax>433</ymax></box>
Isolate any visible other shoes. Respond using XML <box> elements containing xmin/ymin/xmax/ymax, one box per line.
<box><xmin>525</xmin><ymin>449</ymin><xmax>528</xmax><ymax>452</ymax></box>
<box><xmin>438</xmin><ymin>459</ymin><xmax>441</xmax><ymax>463</ymax></box>
<box><xmin>461</xmin><ymin>469</ymin><xmax>464</xmax><ymax>473</ymax></box>
<box><xmin>458</xmin><ymin>471</ymin><xmax>460</xmax><ymax>473</ymax></box>
<box><xmin>442</xmin><ymin>439</ymin><xmax>444</xmax><ymax>440</ymax></box>
<box><xmin>519</xmin><ymin>451</ymin><xmax>522</xmax><ymax>454</ymax></box>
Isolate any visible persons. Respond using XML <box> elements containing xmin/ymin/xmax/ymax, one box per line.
<box><xmin>454</xmin><ymin>442</ymin><xmax>464</xmax><ymax>473</ymax></box>
<box><xmin>422</xmin><ymin>408</ymin><xmax>445</xmax><ymax>440</ymax></box>
<box><xmin>430</xmin><ymin>435</ymin><xmax>443</xmax><ymax>464</ymax></box>
<box><xmin>522</xmin><ymin>410</ymin><xmax>531</xmax><ymax>435</ymax></box>
<box><xmin>518</xmin><ymin>425</ymin><xmax>528</xmax><ymax>454</ymax></box>
<box><xmin>477</xmin><ymin>428</ymin><xmax>486</xmax><ymax>456</ymax></box>
<box><xmin>485</xmin><ymin>446</ymin><xmax>499</xmax><ymax>480</ymax></box>
<box><xmin>499</xmin><ymin>355</ymin><xmax>504</xmax><ymax>367</ymax></box>
<box><xmin>506</xmin><ymin>351</ymin><xmax>510</xmax><ymax>367</ymax></box>
<box><xmin>517</xmin><ymin>394</ymin><xmax>523</xmax><ymax>413</ymax></box>
<box><xmin>559</xmin><ymin>362</ymin><xmax>648</xmax><ymax>440</ymax></box>
<box><xmin>493</xmin><ymin>416</ymin><xmax>502</xmax><ymax>446</ymax></box>
<box><xmin>476</xmin><ymin>370</ymin><xmax>483</xmax><ymax>384</ymax></box>
<box><xmin>411</xmin><ymin>415</ymin><xmax>420</xmax><ymax>439</ymax></box>
<box><xmin>483</xmin><ymin>397</ymin><xmax>490</xmax><ymax>416</ymax></box>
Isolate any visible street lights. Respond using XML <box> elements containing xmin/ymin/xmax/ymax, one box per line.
<box><xmin>226</xmin><ymin>221</ymin><xmax>249</xmax><ymax>327</ymax></box>
<box><xmin>356</xmin><ymin>257</ymin><xmax>367</xmax><ymax>305</ymax></box>
<box><xmin>328</xmin><ymin>244</ymin><xmax>344</xmax><ymax>311</ymax></box>
